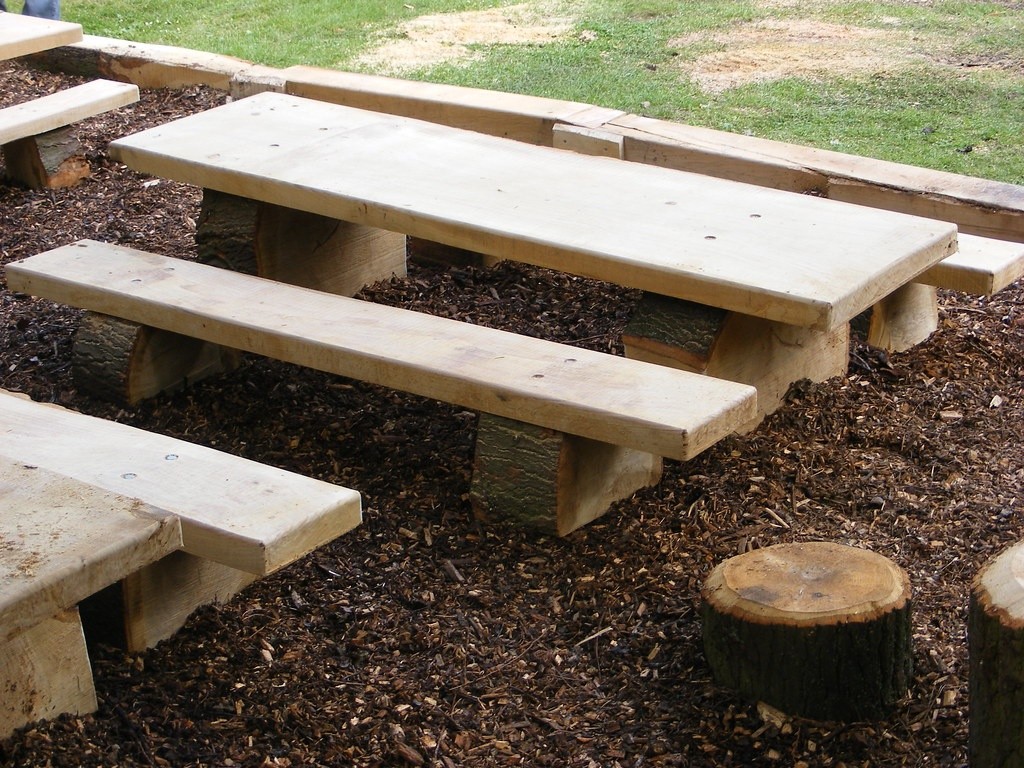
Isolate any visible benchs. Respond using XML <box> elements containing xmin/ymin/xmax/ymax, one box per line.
<box><xmin>0</xmin><ymin>78</ymin><xmax>141</xmax><ymax>190</ymax></box>
<box><xmin>4</xmin><ymin>237</ymin><xmax>760</xmax><ymax>543</ymax></box>
<box><xmin>869</xmin><ymin>234</ymin><xmax>1022</xmax><ymax>355</ymax></box>
<box><xmin>0</xmin><ymin>389</ymin><xmax>361</xmax><ymax>659</ymax></box>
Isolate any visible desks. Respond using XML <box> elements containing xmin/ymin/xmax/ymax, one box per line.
<box><xmin>0</xmin><ymin>465</ymin><xmax>185</xmax><ymax>742</ymax></box>
<box><xmin>109</xmin><ymin>88</ymin><xmax>959</xmax><ymax>437</ymax></box>
<box><xmin>0</xmin><ymin>7</ymin><xmax>82</xmax><ymax>62</ymax></box>
